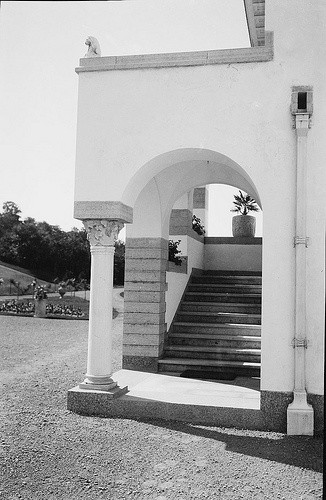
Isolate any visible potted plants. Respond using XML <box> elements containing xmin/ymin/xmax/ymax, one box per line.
<box><xmin>229</xmin><ymin>190</ymin><xmax>260</xmax><ymax>238</ymax></box>
